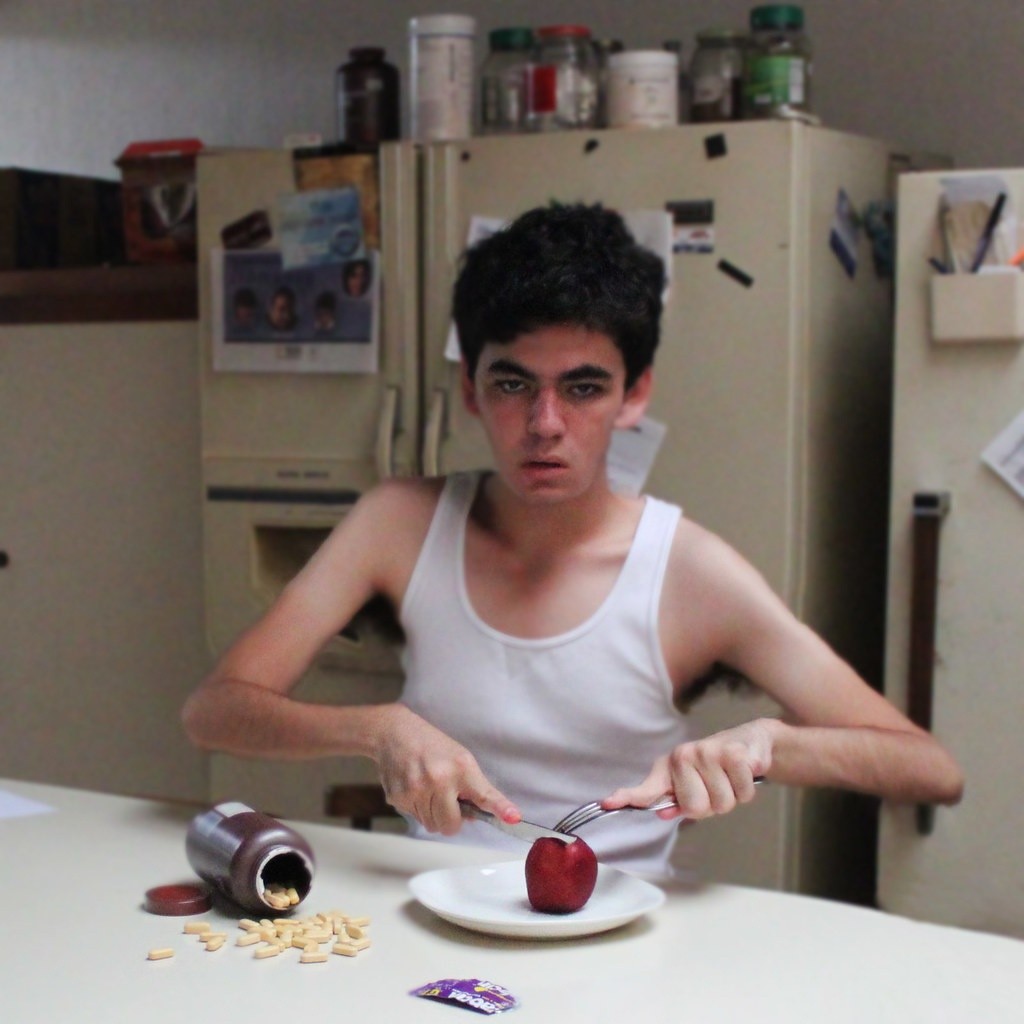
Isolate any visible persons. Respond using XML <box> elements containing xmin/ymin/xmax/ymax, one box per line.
<box><xmin>342</xmin><ymin>259</ymin><xmax>369</xmax><ymax>294</ymax></box>
<box><xmin>266</xmin><ymin>287</ymin><xmax>297</xmax><ymax>328</ymax></box>
<box><xmin>231</xmin><ymin>286</ymin><xmax>262</xmax><ymax>337</ymax></box>
<box><xmin>179</xmin><ymin>203</ymin><xmax>965</xmax><ymax>876</ymax></box>
<box><xmin>311</xmin><ymin>291</ymin><xmax>336</xmax><ymax>328</ymax></box>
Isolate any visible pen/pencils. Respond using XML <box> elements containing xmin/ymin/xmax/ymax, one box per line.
<box><xmin>968</xmin><ymin>192</ymin><xmax>1008</xmax><ymax>271</ymax></box>
<box><xmin>922</xmin><ymin>254</ymin><xmax>948</xmax><ymax>273</ymax></box>
<box><xmin>937</xmin><ymin>192</ymin><xmax>962</xmax><ymax>273</ymax></box>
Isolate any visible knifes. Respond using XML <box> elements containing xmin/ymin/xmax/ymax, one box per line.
<box><xmin>459</xmin><ymin>800</ymin><xmax>578</xmax><ymax>848</ymax></box>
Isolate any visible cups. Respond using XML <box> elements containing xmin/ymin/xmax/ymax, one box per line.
<box><xmin>409</xmin><ymin>13</ymin><xmax>476</xmax><ymax>143</ymax></box>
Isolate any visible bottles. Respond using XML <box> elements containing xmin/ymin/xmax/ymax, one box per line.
<box><xmin>337</xmin><ymin>45</ymin><xmax>403</xmax><ymax>141</ymax></box>
<box><xmin>477</xmin><ymin>5</ymin><xmax>814</xmax><ymax>136</ymax></box>
<box><xmin>185</xmin><ymin>801</ymin><xmax>314</xmax><ymax>919</ymax></box>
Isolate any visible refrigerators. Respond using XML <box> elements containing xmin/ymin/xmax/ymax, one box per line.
<box><xmin>192</xmin><ymin>122</ymin><xmax>953</xmax><ymax>909</ymax></box>
<box><xmin>876</xmin><ymin>166</ymin><xmax>1024</xmax><ymax>941</ymax></box>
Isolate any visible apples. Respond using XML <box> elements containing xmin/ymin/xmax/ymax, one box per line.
<box><xmin>525</xmin><ymin>832</ymin><xmax>598</xmax><ymax>913</ymax></box>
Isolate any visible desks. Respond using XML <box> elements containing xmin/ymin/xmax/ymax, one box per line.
<box><xmin>0</xmin><ymin>774</ymin><xmax>1024</xmax><ymax>1024</ymax></box>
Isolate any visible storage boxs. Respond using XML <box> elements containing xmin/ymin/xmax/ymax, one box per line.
<box><xmin>112</xmin><ymin>139</ymin><xmax>199</xmax><ymax>260</ymax></box>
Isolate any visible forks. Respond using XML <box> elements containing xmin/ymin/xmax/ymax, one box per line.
<box><xmin>552</xmin><ymin>776</ymin><xmax>764</xmax><ymax>836</ymax></box>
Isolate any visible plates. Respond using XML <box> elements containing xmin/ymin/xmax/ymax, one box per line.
<box><xmin>408</xmin><ymin>860</ymin><xmax>665</xmax><ymax>942</ymax></box>
